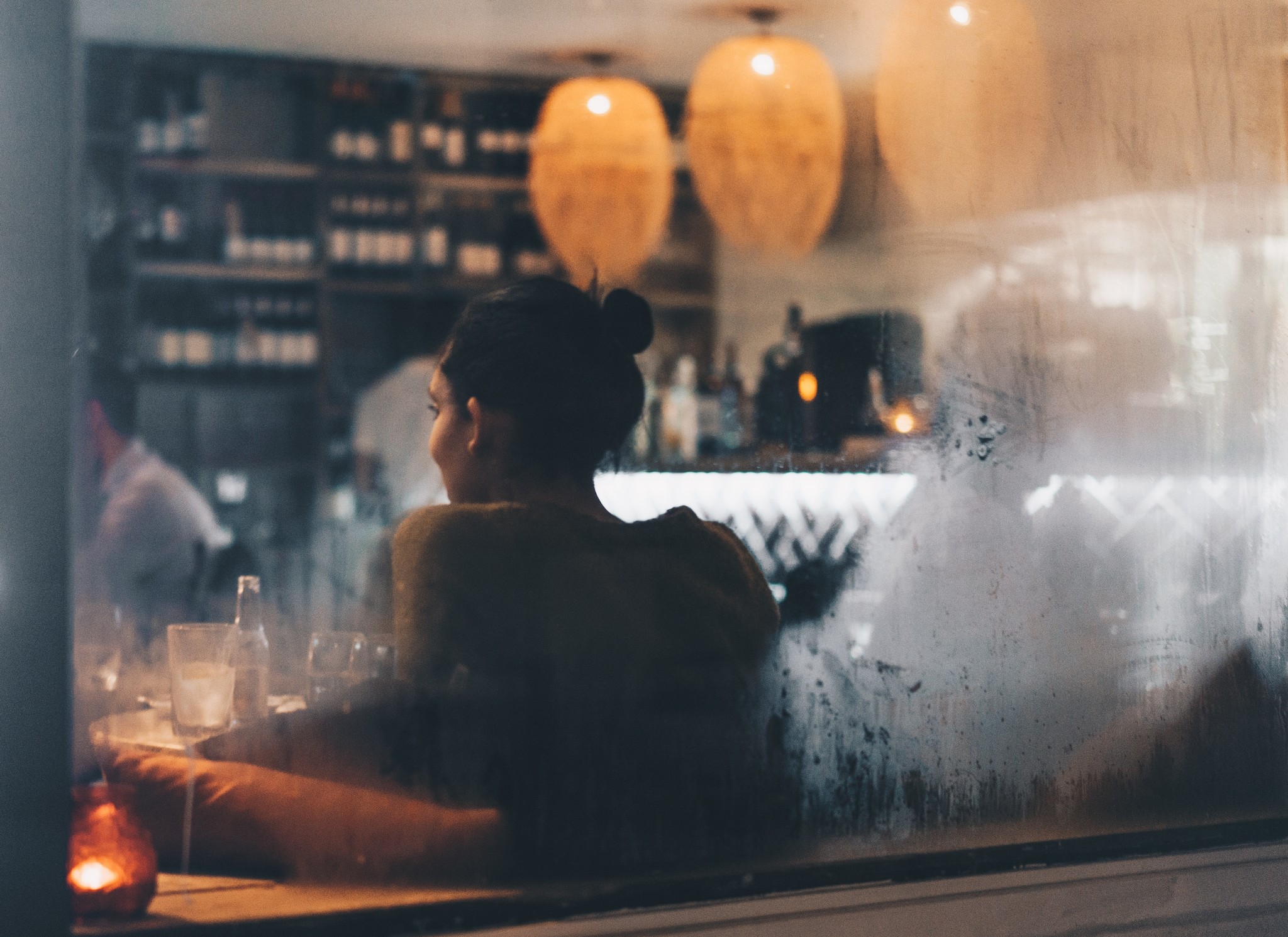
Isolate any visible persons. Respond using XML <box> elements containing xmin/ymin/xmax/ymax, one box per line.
<box><xmin>354</xmin><ymin>299</ymin><xmax>459</xmax><ymax>527</ymax></box>
<box><xmin>392</xmin><ymin>267</ymin><xmax>782</xmax><ymax>680</ymax></box>
<box><xmin>91</xmin><ymin>402</ymin><xmax>231</xmax><ymax>607</ymax></box>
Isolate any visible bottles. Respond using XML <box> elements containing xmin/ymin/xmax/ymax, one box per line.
<box><xmin>628</xmin><ymin>339</ymin><xmax>749</xmax><ymax>460</ymax></box>
<box><xmin>133</xmin><ymin>171</ymin><xmax>317</xmax><ymax>263</ymax></box>
<box><xmin>326</xmin><ymin>68</ymin><xmax>562</xmax><ymax>278</ymax></box>
<box><xmin>231</xmin><ymin>575</ymin><xmax>269</xmax><ymax>723</ymax></box>
<box><xmin>137</xmin><ymin>278</ymin><xmax>322</xmax><ymax>382</ymax></box>
<box><xmin>133</xmin><ymin>57</ymin><xmax>209</xmax><ymax>158</ymax></box>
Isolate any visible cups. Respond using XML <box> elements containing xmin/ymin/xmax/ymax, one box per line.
<box><xmin>69</xmin><ymin>604</ymin><xmax>121</xmax><ymax>693</ymax></box>
<box><xmin>303</xmin><ymin>632</ymin><xmax>367</xmax><ymax>705</ymax></box>
<box><xmin>167</xmin><ymin>624</ymin><xmax>237</xmax><ymax>738</ymax></box>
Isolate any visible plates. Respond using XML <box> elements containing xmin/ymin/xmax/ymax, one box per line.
<box><xmin>148</xmin><ymin>698</ymin><xmax>285</xmax><ymax>719</ymax></box>
